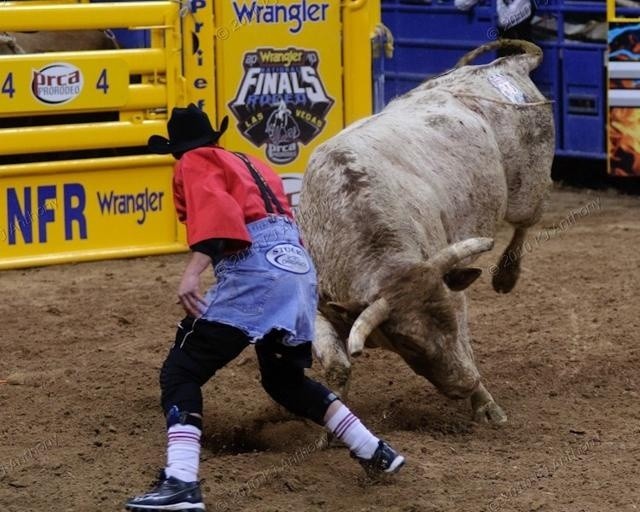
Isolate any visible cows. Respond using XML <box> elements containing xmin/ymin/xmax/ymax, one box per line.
<box><xmin>291</xmin><ymin>37</ymin><xmax>557</xmax><ymax>453</ymax></box>
<box><xmin>0</xmin><ymin>29</ymin><xmax>130</xmax><ymax>167</ymax></box>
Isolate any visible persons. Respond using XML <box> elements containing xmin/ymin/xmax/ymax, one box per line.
<box><xmin>122</xmin><ymin>100</ymin><xmax>409</xmax><ymax>511</ymax></box>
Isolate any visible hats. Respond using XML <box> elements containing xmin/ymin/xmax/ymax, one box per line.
<box><xmin>144</xmin><ymin>101</ymin><xmax>237</xmax><ymax>155</ymax></box>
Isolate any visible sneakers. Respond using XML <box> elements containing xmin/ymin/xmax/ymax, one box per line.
<box><xmin>121</xmin><ymin>473</ymin><xmax>210</xmax><ymax>512</ymax></box>
<box><xmin>345</xmin><ymin>434</ymin><xmax>409</xmax><ymax>483</ymax></box>
